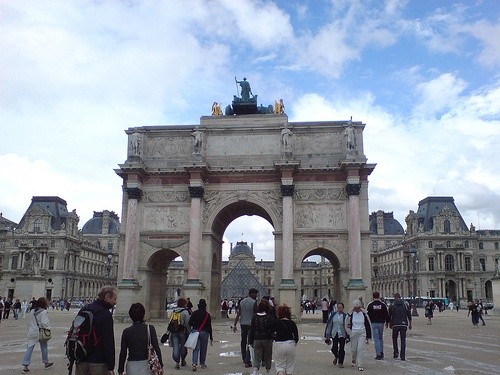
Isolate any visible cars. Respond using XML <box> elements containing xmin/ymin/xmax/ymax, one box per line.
<box><xmin>70</xmin><ymin>300</ymin><xmax>85</xmax><ymax>308</ymax></box>
<box><xmin>166</xmin><ymin>303</ymin><xmax>177</xmax><ymax>309</ymax></box>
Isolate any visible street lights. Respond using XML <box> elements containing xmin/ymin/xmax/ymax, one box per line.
<box><xmin>402</xmin><ymin>244</ymin><xmax>419</xmax><ymax>316</ymax></box>
<box><xmin>107</xmin><ymin>250</ymin><xmax>113</xmax><ymax>285</ymax></box>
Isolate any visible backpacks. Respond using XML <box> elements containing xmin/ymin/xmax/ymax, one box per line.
<box><xmin>167</xmin><ymin>309</ymin><xmax>187</xmax><ymax>335</ymax></box>
<box><xmin>63</xmin><ymin>305</ymin><xmax>98</xmax><ymax>368</ymax></box>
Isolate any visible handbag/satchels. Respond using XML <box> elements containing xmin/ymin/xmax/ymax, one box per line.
<box><xmin>38</xmin><ymin>328</ymin><xmax>52</xmax><ymax>341</ymax></box>
<box><xmin>160</xmin><ymin>330</ymin><xmax>171</xmax><ymax>343</ymax></box>
<box><xmin>147</xmin><ymin>324</ymin><xmax>164</xmax><ymax>375</ymax></box>
<box><xmin>184</xmin><ymin>331</ymin><xmax>200</xmax><ymax>349</ymax></box>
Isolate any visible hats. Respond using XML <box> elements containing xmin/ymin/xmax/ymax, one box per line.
<box><xmin>197</xmin><ymin>299</ymin><xmax>207</xmax><ymax>307</ymax></box>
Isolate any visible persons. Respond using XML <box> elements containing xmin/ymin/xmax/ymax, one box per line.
<box><xmin>131</xmin><ymin>130</ymin><xmax>140</xmax><ymax>154</ymax></box>
<box><xmin>46</xmin><ymin>291</ymin><xmax>70</xmax><ymax>314</ymax></box>
<box><xmin>304</xmin><ymin>298</ymin><xmax>316</xmax><ymax>314</ymax></box>
<box><xmin>282</xmin><ymin>125</ymin><xmax>292</xmax><ymax>148</ymax></box>
<box><xmin>171</xmin><ymin>298</ymin><xmax>214</xmax><ymax>371</ymax></box>
<box><xmin>359</xmin><ymin>296</ymin><xmax>364</xmax><ymax>308</ymax></box>
<box><xmin>22</xmin><ymin>297</ymin><xmax>54</xmax><ymax>373</ymax></box>
<box><xmin>191</xmin><ymin>127</ymin><xmax>203</xmax><ymax>153</ymax></box>
<box><xmin>234</xmin><ymin>289</ymin><xmax>299</xmax><ymax>375</ymax></box>
<box><xmin>321</xmin><ymin>298</ymin><xmax>337</xmax><ymax>323</ymax></box>
<box><xmin>118</xmin><ymin>303</ymin><xmax>164</xmax><ymax>375</ymax></box>
<box><xmin>467</xmin><ymin>299</ymin><xmax>486</xmax><ymax>326</ymax></box>
<box><xmin>345</xmin><ymin>122</ymin><xmax>358</xmax><ymax>149</ymax></box>
<box><xmin>436</xmin><ymin>300</ymin><xmax>460</xmax><ymax>311</ymax></box>
<box><xmin>235</xmin><ymin>76</ymin><xmax>254</xmax><ymax>99</ymax></box>
<box><xmin>324</xmin><ymin>300</ymin><xmax>372</xmax><ymax>370</ymax></box>
<box><xmin>0</xmin><ymin>296</ymin><xmax>37</xmax><ymax>321</ymax></box>
<box><xmin>220</xmin><ymin>299</ymin><xmax>234</xmax><ymax>318</ymax></box>
<box><xmin>425</xmin><ymin>301</ymin><xmax>434</xmax><ymax>325</ymax></box>
<box><xmin>72</xmin><ymin>285</ymin><xmax>119</xmax><ymax>375</ymax></box>
<box><xmin>79</xmin><ymin>298</ymin><xmax>92</xmax><ymax>308</ymax></box>
<box><xmin>367</xmin><ymin>290</ymin><xmax>412</xmax><ymax>359</ymax></box>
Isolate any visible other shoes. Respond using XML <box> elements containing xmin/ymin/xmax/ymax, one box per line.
<box><xmin>181</xmin><ymin>361</ymin><xmax>186</xmax><ymax>366</ymax></box>
<box><xmin>338</xmin><ymin>364</ymin><xmax>344</xmax><ymax>368</ymax></box>
<box><xmin>352</xmin><ymin>362</ymin><xmax>356</xmax><ymax>367</ymax></box>
<box><xmin>175</xmin><ymin>364</ymin><xmax>180</xmax><ymax>369</ymax></box>
<box><xmin>201</xmin><ymin>364</ymin><xmax>207</xmax><ymax>368</ymax></box>
<box><xmin>45</xmin><ymin>362</ymin><xmax>54</xmax><ymax>367</ymax></box>
<box><xmin>375</xmin><ymin>355</ymin><xmax>384</xmax><ymax>360</ymax></box>
<box><xmin>22</xmin><ymin>369</ymin><xmax>30</xmax><ymax>373</ymax></box>
<box><xmin>192</xmin><ymin>366</ymin><xmax>197</xmax><ymax>371</ymax></box>
<box><xmin>333</xmin><ymin>356</ymin><xmax>338</xmax><ymax>365</ymax></box>
<box><xmin>358</xmin><ymin>367</ymin><xmax>364</xmax><ymax>371</ymax></box>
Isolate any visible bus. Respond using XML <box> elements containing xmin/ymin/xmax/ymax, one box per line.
<box><xmin>384</xmin><ymin>296</ymin><xmax>448</xmax><ymax>308</ymax></box>
